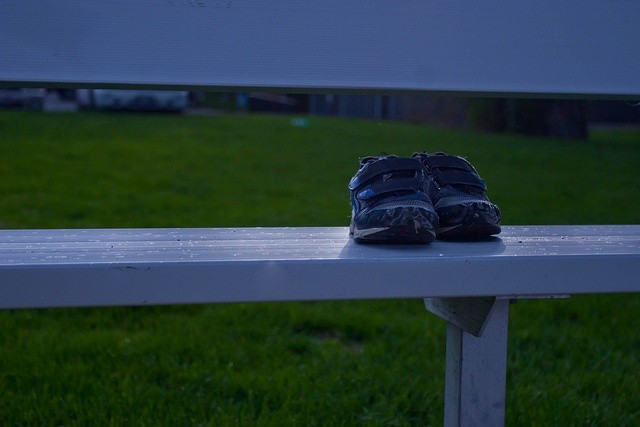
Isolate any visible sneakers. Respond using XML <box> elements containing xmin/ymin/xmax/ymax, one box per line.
<box><xmin>412</xmin><ymin>152</ymin><xmax>501</xmax><ymax>241</ymax></box>
<box><xmin>349</xmin><ymin>156</ymin><xmax>440</xmax><ymax>244</ymax></box>
